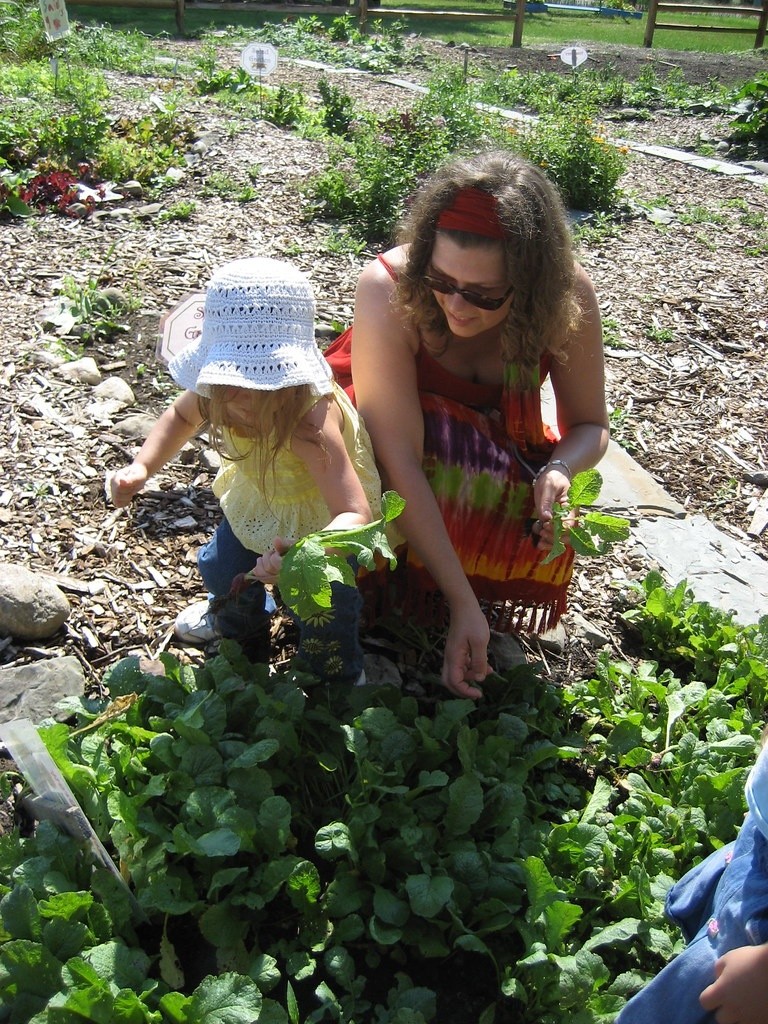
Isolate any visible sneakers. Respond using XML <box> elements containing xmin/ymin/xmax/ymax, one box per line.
<box><xmin>174</xmin><ymin>598</ymin><xmax>222</xmax><ymax>644</ymax></box>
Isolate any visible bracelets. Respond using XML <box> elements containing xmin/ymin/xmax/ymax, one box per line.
<box><xmin>533</xmin><ymin>460</ymin><xmax>572</xmax><ymax>488</ymax></box>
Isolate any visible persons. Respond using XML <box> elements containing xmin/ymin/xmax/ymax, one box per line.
<box><xmin>613</xmin><ymin>737</ymin><xmax>768</xmax><ymax>1024</ymax></box>
<box><xmin>324</xmin><ymin>155</ymin><xmax>610</xmax><ymax>700</ymax></box>
<box><xmin>109</xmin><ymin>257</ymin><xmax>403</xmax><ymax>702</ymax></box>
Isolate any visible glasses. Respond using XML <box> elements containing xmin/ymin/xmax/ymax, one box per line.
<box><xmin>421</xmin><ymin>261</ymin><xmax>515</xmax><ymax>311</ymax></box>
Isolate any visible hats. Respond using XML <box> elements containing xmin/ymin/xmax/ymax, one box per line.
<box><xmin>169</xmin><ymin>258</ymin><xmax>334</xmax><ymax>400</ymax></box>
